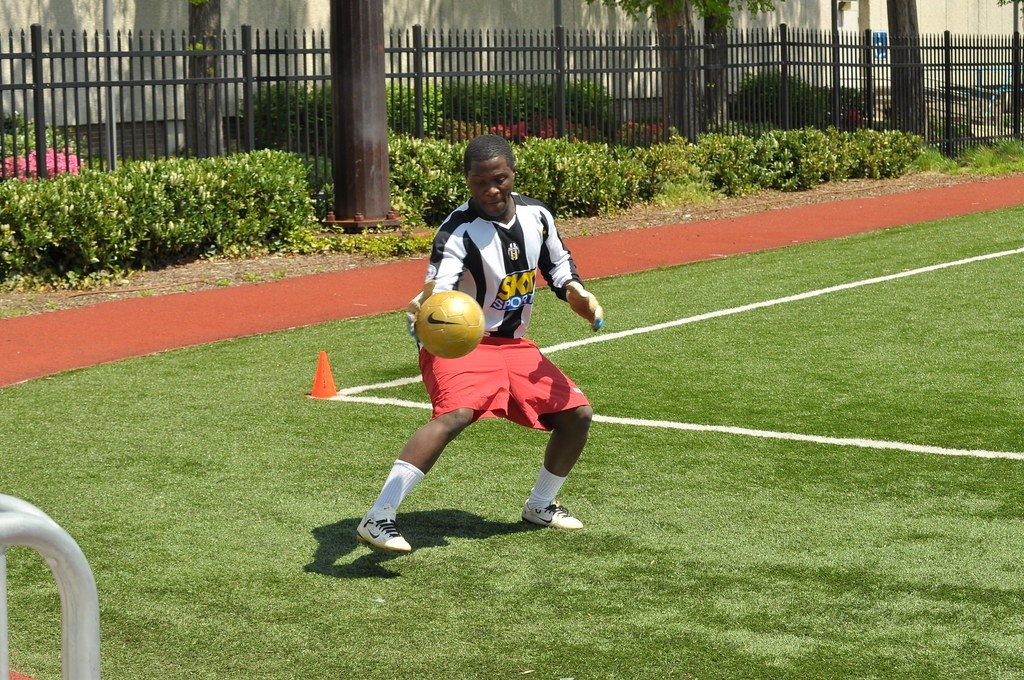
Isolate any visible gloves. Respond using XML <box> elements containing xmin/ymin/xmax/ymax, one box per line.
<box><xmin>403</xmin><ymin>280</ymin><xmax>436</xmax><ymax>341</ymax></box>
<box><xmin>567</xmin><ymin>280</ymin><xmax>603</xmax><ymax>332</ymax></box>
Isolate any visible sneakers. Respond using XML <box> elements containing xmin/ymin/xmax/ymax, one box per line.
<box><xmin>521</xmin><ymin>501</ymin><xmax>583</xmax><ymax>529</ymax></box>
<box><xmin>356</xmin><ymin>503</ymin><xmax>412</xmax><ymax>553</ymax></box>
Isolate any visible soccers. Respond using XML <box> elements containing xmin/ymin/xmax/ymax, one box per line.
<box><xmin>416</xmin><ymin>290</ymin><xmax>486</xmax><ymax>359</ymax></box>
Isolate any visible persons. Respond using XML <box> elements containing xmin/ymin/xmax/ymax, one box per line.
<box><xmin>355</xmin><ymin>135</ymin><xmax>604</xmax><ymax>553</ymax></box>
